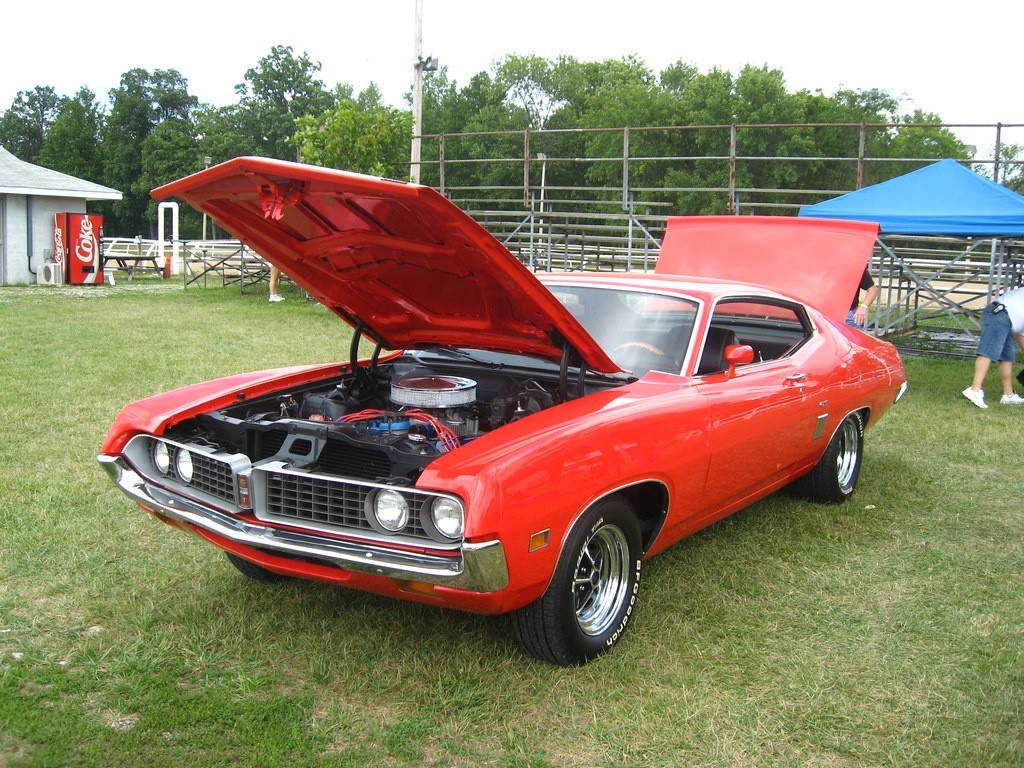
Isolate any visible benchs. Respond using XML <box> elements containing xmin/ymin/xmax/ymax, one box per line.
<box><xmin>118</xmin><ymin>265</ymin><xmax>165</xmax><ymax>272</ymax></box>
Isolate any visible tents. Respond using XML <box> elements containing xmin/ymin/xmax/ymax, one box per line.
<box><xmin>795</xmin><ymin>158</ymin><xmax>1024</xmax><ymax>361</ymax></box>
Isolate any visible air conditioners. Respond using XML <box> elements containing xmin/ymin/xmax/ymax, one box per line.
<box><xmin>37</xmin><ymin>263</ymin><xmax>63</xmax><ymax>285</ymax></box>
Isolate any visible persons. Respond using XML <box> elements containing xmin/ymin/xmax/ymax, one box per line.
<box><xmin>961</xmin><ymin>283</ymin><xmax>1024</xmax><ymax>409</ymax></box>
<box><xmin>847</xmin><ymin>267</ymin><xmax>878</xmax><ymax>331</ymax></box>
<box><xmin>267</xmin><ymin>262</ymin><xmax>286</xmax><ymax>302</ymax></box>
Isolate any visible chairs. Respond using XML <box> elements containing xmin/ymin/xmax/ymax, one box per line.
<box><xmin>666</xmin><ymin>325</ymin><xmax>741</xmax><ymax>375</ymax></box>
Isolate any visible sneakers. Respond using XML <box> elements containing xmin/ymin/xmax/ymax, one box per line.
<box><xmin>962</xmin><ymin>387</ymin><xmax>988</xmax><ymax>408</ymax></box>
<box><xmin>269</xmin><ymin>294</ymin><xmax>285</xmax><ymax>302</ymax></box>
<box><xmin>1000</xmin><ymin>390</ymin><xmax>1024</xmax><ymax>404</ymax></box>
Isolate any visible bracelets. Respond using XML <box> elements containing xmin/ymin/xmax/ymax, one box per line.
<box><xmin>859</xmin><ymin>302</ymin><xmax>870</xmax><ymax>309</ymax></box>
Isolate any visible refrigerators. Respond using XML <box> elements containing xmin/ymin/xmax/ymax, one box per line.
<box><xmin>55</xmin><ymin>213</ymin><xmax>104</xmax><ymax>285</ymax></box>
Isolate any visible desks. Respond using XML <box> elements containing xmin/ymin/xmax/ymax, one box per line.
<box><xmin>103</xmin><ymin>255</ymin><xmax>164</xmax><ymax>281</ymax></box>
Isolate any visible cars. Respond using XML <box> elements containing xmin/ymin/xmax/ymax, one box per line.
<box><xmin>100</xmin><ymin>159</ymin><xmax>911</xmax><ymax>667</ymax></box>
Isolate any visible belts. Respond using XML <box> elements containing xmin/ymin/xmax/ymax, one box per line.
<box><xmin>992</xmin><ymin>301</ymin><xmax>1008</xmax><ymax>314</ymax></box>
